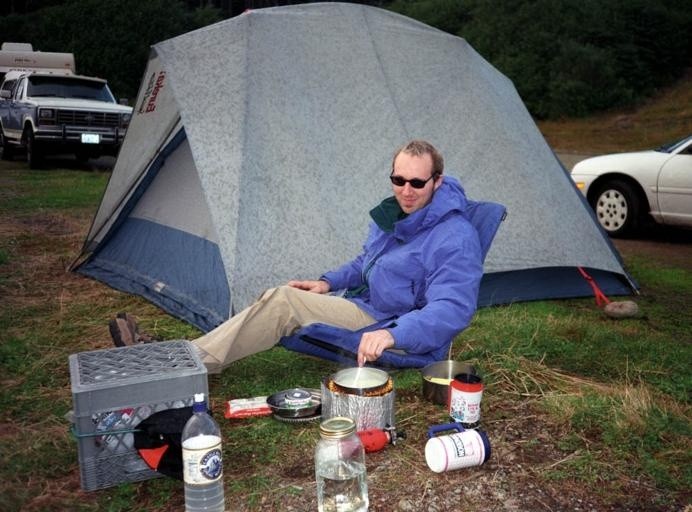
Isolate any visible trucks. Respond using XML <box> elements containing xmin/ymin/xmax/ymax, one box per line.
<box><xmin>0</xmin><ymin>41</ymin><xmax>77</xmax><ymax>86</ymax></box>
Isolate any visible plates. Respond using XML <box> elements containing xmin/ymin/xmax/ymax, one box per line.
<box><xmin>331</xmin><ymin>366</ymin><xmax>390</xmax><ymax>392</ymax></box>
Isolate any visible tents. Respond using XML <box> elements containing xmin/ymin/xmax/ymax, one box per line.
<box><xmin>69</xmin><ymin>3</ymin><xmax>643</xmax><ymax>342</ymax></box>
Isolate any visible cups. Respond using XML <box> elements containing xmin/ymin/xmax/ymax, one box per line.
<box><xmin>445</xmin><ymin>374</ymin><xmax>484</xmax><ymax>431</ymax></box>
<box><xmin>425</xmin><ymin>422</ymin><xmax>490</xmax><ymax>473</ymax></box>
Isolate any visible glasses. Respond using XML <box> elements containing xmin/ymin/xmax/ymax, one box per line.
<box><xmin>389</xmin><ymin>169</ymin><xmax>441</xmax><ymax>190</ymax></box>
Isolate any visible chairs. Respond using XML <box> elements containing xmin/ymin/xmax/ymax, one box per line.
<box><xmin>284</xmin><ymin>199</ymin><xmax>508</xmax><ymax>372</ymax></box>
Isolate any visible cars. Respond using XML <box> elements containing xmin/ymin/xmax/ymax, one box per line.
<box><xmin>570</xmin><ymin>135</ymin><xmax>691</xmax><ymax>236</ymax></box>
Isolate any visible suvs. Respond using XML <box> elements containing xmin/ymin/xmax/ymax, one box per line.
<box><xmin>0</xmin><ymin>71</ymin><xmax>136</xmax><ymax>168</ymax></box>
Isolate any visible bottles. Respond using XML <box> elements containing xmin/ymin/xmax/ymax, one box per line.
<box><xmin>182</xmin><ymin>392</ymin><xmax>226</xmax><ymax>511</ymax></box>
<box><xmin>315</xmin><ymin>416</ymin><xmax>370</xmax><ymax>511</ymax></box>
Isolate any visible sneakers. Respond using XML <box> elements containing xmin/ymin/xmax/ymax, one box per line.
<box><xmin>107</xmin><ymin>309</ymin><xmax>162</xmax><ymax>347</ymax></box>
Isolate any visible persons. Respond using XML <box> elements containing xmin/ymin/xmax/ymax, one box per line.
<box><xmin>110</xmin><ymin>140</ymin><xmax>506</xmax><ymax>382</ymax></box>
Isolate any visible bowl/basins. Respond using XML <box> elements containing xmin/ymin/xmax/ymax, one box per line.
<box><xmin>421</xmin><ymin>359</ymin><xmax>478</xmax><ymax>406</ymax></box>
<box><xmin>266</xmin><ymin>388</ymin><xmax>322</xmax><ymax>418</ymax></box>
